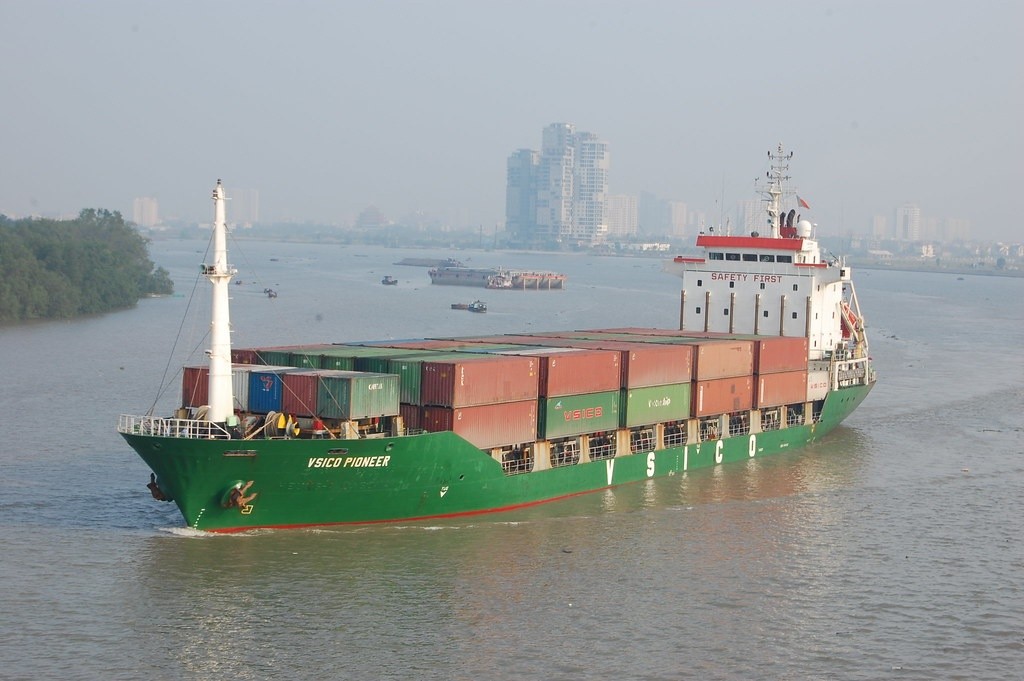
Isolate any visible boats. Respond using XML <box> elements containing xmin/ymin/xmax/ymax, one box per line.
<box><xmin>451</xmin><ymin>299</ymin><xmax>487</xmax><ymax>314</ymax></box>
<box><xmin>402</xmin><ymin>254</ymin><xmax>567</xmax><ymax>290</ymax></box>
<box><xmin>117</xmin><ymin>142</ymin><xmax>877</xmax><ymax>538</ymax></box>
<box><xmin>382</xmin><ymin>275</ymin><xmax>398</xmax><ymax>285</ymax></box>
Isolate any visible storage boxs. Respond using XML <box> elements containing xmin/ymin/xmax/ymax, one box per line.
<box><xmin>181</xmin><ymin>328</ymin><xmax>810</xmax><ymax>450</ymax></box>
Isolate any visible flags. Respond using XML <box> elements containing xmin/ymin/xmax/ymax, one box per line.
<box><xmin>797</xmin><ymin>197</ymin><xmax>808</xmax><ymax>210</ymax></box>
